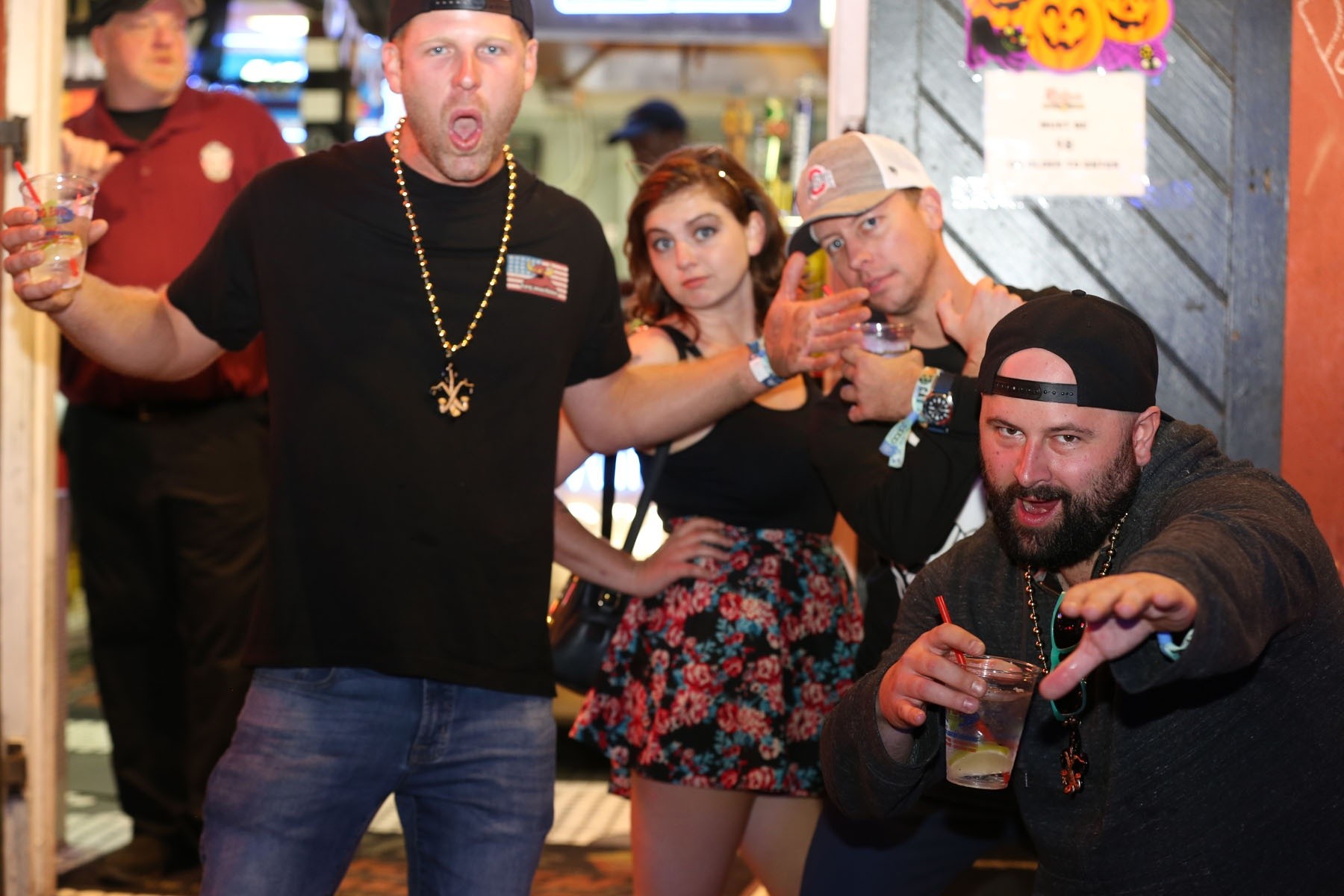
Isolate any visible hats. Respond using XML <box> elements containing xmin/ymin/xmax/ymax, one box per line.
<box><xmin>608</xmin><ymin>102</ymin><xmax>686</xmax><ymax>142</ymax></box>
<box><xmin>387</xmin><ymin>0</ymin><xmax>533</xmax><ymax>41</ymax></box>
<box><xmin>787</xmin><ymin>130</ymin><xmax>932</xmax><ymax>256</ymax></box>
<box><xmin>88</xmin><ymin>0</ymin><xmax>206</xmax><ymax>26</ymax></box>
<box><xmin>976</xmin><ymin>289</ymin><xmax>1176</xmax><ymax>422</ymax></box>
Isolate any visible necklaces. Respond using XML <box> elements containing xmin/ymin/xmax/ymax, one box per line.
<box><xmin>1023</xmin><ymin>510</ymin><xmax>1129</xmax><ymax>798</ymax></box>
<box><xmin>391</xmin><ymin>117</ymin><xmax>521</xmax><ymax>418</ymax></box>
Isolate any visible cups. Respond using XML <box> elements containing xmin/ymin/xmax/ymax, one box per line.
<box><xmin>18</xmin><ymin>172</ymin><xmax>99</xmax><ymax>290</ymax></box>
<box><xmin>859</xmin><ymin>322</ymin><xmax>916</xmax><ymax>358</ymax></box>
<box><xmin>945</xmin><ymin>652</ymin><xmax>1043</xmax><ymax>791</ymax></box>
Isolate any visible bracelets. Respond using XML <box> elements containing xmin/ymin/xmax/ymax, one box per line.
<box><xmin>1156</xmin><ymin>628</ymin><xmax>1194</xmax><ymax>659</ymax></box>
<box><xmin>879</xmin><ymin>366</ymin><xmax>942</xmax><ymax>468</ymax></box>
<box><xmin>748</xmin><ymin>336</ymin><xmax>791</xmax><ymax>390</ymax></box>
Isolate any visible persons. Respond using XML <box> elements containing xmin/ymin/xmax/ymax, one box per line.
<box><xmin>548</xmin><ymin>143</ymin><xmax>870</xmax><ymax>896</ymax></box>
<box><xmin>793</xmin><ymin>130</ymin><xmax>1054</xmax><ymax>896</ymax></box>
<box><xmin>53</xmin><ymin>0</ymin><xmax>298</xmax><ymax>896</ymax></box>
<box><xmin>812</xmin><ymin>288</ymin><xmax>1344</xmax><ymax>896</ymax></box>
<box><xmin>605</xmin><ymin>94</ymin><xmax>697</xmax><ymax>181</ymax></box>
<box><xmin>1</xmin><ymin>0</ymin><xmax>875</xmax><ymax>896</ymax></box>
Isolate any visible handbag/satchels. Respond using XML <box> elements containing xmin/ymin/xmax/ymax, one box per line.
<box><xmin>554</xmin><ymin>577</ymin><xmax>631</xmax><ymax>695</ymax></box>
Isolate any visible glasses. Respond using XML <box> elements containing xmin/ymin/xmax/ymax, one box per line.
<box><xmin>1050</xmin><ymin>591</ymin><xmax>1087</xmax><ymax>720</ymax></box>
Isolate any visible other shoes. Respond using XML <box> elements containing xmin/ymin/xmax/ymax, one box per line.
<box><xmin>95</xmin><ymin>822</ymin><xmax>204</xmax><ymax>880</ymax></box>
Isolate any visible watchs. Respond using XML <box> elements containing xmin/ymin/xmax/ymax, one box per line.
<box><xmin>920</xmin><ymin>370</ymin><xmax>953</xmax><ymax>434</ymax></box>
